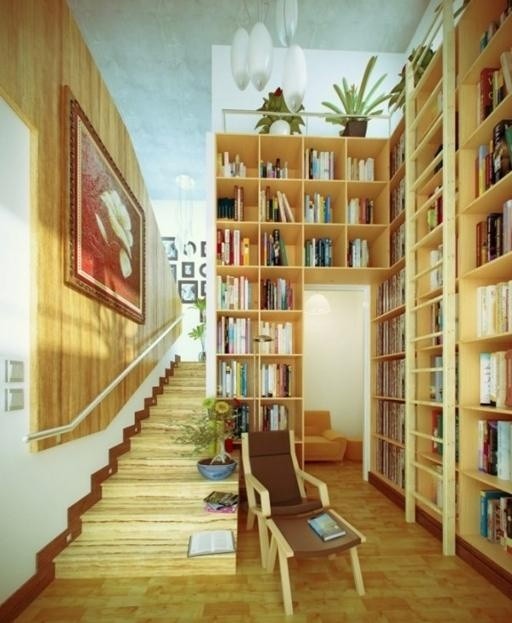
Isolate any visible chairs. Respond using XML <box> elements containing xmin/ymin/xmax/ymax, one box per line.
<box><xmin>304</xmin><ymin>410</ymin><xmax>347</xmax><ymax>466</ymax></box>
<box><xmin>240</xmin><ymin>428</ymin><xmax>366</xmax><ymax>617</ymax></box>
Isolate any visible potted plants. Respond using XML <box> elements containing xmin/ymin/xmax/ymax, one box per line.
<box><xmin>320</xmin><ymin>55</ymin><xmax>392</xmax><ymax>137</ymax></box>
<box><xmin>254</xmin><ymin>87</ymin><xmax>305</xmax><ymax>135</ymax></box>
<box><xmin>165</xmin><ymin>397</ymin><xmax>244</xmax><ymax>481</ymax></box>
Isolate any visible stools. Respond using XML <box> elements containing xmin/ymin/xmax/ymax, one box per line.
<box><xmin>344</xmin><ymin>436</ymin><xmax>363</xmax><ymax>463</ymax></box>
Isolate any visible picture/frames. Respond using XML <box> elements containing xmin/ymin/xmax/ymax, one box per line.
<box><xmin>159</xmin><ymin>236</ymin><xmax>207</xmax><ymax>303</ymax></box>
<box><xmin>61</xmin><ymin>84</ymin><xmax>145</xmax><ymax>324</ymax></box>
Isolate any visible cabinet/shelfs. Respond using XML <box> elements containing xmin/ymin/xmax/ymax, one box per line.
<box><xmin>363</xmin><ymin>0</ymin><xmax>512</xmax><ymax>599</ymax></box>
<box><xmin>204</xmin><ymin>133</ymin><xmax>390</xmax><ymax>468</ymax></box>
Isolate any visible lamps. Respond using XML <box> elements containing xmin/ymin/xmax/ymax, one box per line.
<box><xmin>230</xmin><ymin>0</ymin><xmax>308</xmax><ymax>113</ymax></box>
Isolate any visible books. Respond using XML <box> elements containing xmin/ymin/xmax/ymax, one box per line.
<box><xmin>427</xmin><ymin>185</ymin><xmax>443</xmax><ymax>234</ymax></box>
<box><xmin>480</xmin><ymin>350</ymin><xmax>512</xmax><ymax>409</ymax></box>
<box><xmin>477</xmin><ymin>419</ymin><xmax>512</xmax><ymax>480</ymax></box>
<box><xmin>187</xmin><ymin>527</ymin><xmax>238</xmax><ymax>557</ymax></box>
<box><xmin>308</xmin><ymin>510</ymin><xmax>347</xmax><ymax>541</ymax></box>
<box><xmin>476</xmin><ymin>51</ymin><xmax>512</xmax><ymax>124</ymax></box>
<box><xmin>374</xmin><ymin>222</ymin><xmax>406</xmax><ymax>490</ymax></box>
<box><xmin>217</xmin><ymin>272</ymin><xmax>297</xmax><ymax>440</ymax></box>
<box><xmin>431</xmin><ymin>352</ymin><xmax>458</xmax><ymax>403</ymax></box>
<box><xmin>431</xmin><ymin>293</ymin><xmax>457</xmax><ymax>345</ymax></box>
<box><xmin>432</xmin><ymin>408</ymin><xmax>459</xmax><ymax>462</ymax></box>
<box><xmin>202</xmin><ymin>489</ymin><xmax>239</xmax><ymax>513</ymax></box>
<box><xmin>476</xmin><ymin>280</ymin><xmax>512</xmax><ymax>338</ymax></box>
<box><xmin>389</xmin><ymin>177</ymin><xmax>406</xmax><ymax>223</ymax></box>
<box><xmin>214</xmin><ymin>145</ymin><xmax>377</xmax><ymax>268</ymax></box>
<box><xmin>474</xmin><ymin>119</ymin><xmax>512</xmax><ymax>198</ymax></box>
<box><xmin>390</xmin><ymin>129</ymin><xmax>405</xmax><ymax>180</ymax></box>
<box><xmin>429</xmin><ymin>247</ymin><xmax>443</xmax><ymax>288</ymax></box>
<box><xmin>479</xmin><ymin>489</ymin><xmax>512</xmax><ymax>550</ymax></box>
<box><xmin>475</xmin><ymin>199</ymin><xmax>512</xmax><ymax>268</ymax></box>
<box><xmin>475</xmin><ymin>0</ymin><xmax>512</xmax><ymax>54</ymax></box>
